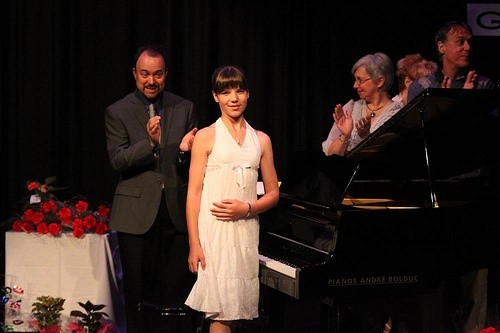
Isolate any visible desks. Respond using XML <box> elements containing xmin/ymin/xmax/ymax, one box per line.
<box><xmin>5</xmin><ymin>230</ymin><xmax>131</xmax><ymax>333</ymax></box>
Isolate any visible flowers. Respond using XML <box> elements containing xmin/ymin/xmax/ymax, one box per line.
<box><xmin>2</xmin><ymin>176</ymin><xmax>111</xmax><ymax>238</ymax></box>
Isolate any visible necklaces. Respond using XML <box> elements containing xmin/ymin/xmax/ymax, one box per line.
<box><xmin>366</xmin><ymin>104</ymin><xmax>383</xmax><ymax>117</ymax></box>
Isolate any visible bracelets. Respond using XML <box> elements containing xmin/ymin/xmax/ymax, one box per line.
<box><xmin>242</xmin><ymin>203</ymin><xmax>252</xmax><ymax>219</ymax></box>
<box><xmin>338</xmin><ymin>134</ymin><xmax>352</xmax><ymax>144</ymax></box>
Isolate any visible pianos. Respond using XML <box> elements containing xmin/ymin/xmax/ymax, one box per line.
<box><xmin>256</xmin><ymin>86</ymin><xmax>500</xmax><ymax>333</ymax></box>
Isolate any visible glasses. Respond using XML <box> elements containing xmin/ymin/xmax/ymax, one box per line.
<box><xmin>354</xmin><ymin>76</ymin><xmax>373</xmax><ymax>84</ymax></box>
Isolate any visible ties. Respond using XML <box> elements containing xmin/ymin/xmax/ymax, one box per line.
<box><xmin>148</xmin><ymin>104</ymin><xmax>162</xmax><ymax>146</ymax></box>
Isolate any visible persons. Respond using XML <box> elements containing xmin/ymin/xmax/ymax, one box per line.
<box><xmin>390</xmin><ymin>53</ymin><xmax>438</xmax><ymax>105</ymax></box>
<box><xmin>105</xmin><ymin>46</ymin><xmax>206</xmax><ymax>333</ymax></box>
<box><xmin>183</xmin><ymin>66</ymin><xmax>280</xmax><ymax>333</ymax></box>
<box><xmin>321</xmin><ymin>53</ymin><xmax>403</xmax><ymax>157</ymax></box>
<box><xmin>407</xmin><ymin>21</ymin><xmax>495</xmax><ymax>103</ymax></box>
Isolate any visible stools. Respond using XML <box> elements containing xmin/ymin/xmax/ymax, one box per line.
<box><xmin>135</xmin><ymin>295</ymin><xmax>203</xmax><ymax>333</ymax></box>
